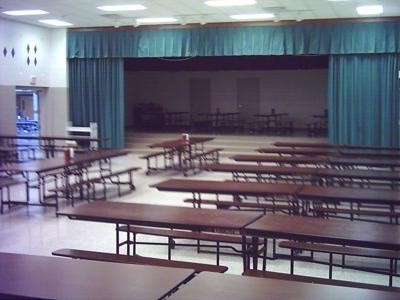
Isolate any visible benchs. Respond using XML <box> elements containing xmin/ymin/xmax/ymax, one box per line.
<box><xmin>140</xmin><ymin>148</ymin><xmax>223</xmax><ymax>176</ymax></box>
<box><xmin>52</xmin><ymin>226</ymin><xmax>399</xmax><ymax>293</ymax></box>
<box><xmin>232</xmin><ymin>172</ymin><xmax>398</xmax><ymax>188</ymax></box>
<box><xmin>49</xmin><ymin>165</ymin><xmax>141</xmax><ymax>216</ymax></box>
<box><xmin>183</xmin><ymin>197</ymin><xmax>395</xmax><ymax>223</ymax></box>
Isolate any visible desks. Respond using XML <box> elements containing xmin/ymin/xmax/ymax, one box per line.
<box><xmin>2</xmin><ymin>252</ymin><xmax>394</xmax><ymax>299</ymax></box>
<box><xmin>3</xmin><ymin>133</ymin><xmax>127</xmax><ymax>203</ymax></box>
<box><xmin>150</xmin><ymin>178</ymin><xmax>400</xmax><ymax>204</ymax></box>
<box><xmin>57</xmin><ymin>200</ymin><xmax>394</xmax><ymax>285</ymax></box>
<box><xmin>203</xmin><ymin>142</ymin><xmax>399</xmax><ymax>178</ymax></box>
<box><xmin>149</xmin><ymin>135</ymin><xmax>214</xmax><ymax>170</ymax></box>
